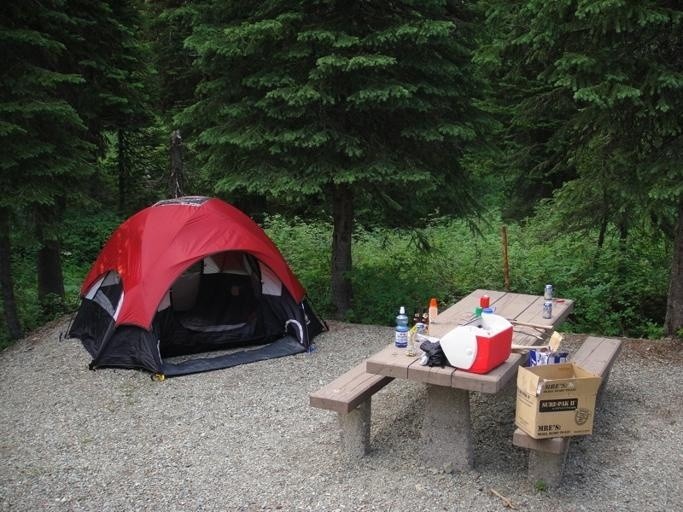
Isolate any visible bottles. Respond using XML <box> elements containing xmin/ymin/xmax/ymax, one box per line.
<box><xmin>475</xmin><ymin>295</ymin><xmax>495</xmax><ymax>329</ymax></box>
<box><xmin>427</xmin><ymin>298</ymin><xmax>438</xmax><ymax>325</ymax></box>
<box><xmin>413</xmin><ymin>306</ymin><xmax>429</xmax><ymax>331</ymax></box>
<box><xmin>394</xmin><ymin>306</ymin><xmax>408</xmax><ymax>348</ymax></box>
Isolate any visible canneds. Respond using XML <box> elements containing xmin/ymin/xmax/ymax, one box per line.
<box><xmin>544</xmin><ymin>285</ymin><xmax>553</xmax><ymax>300</ymax></box>
<box><xmin>543</xmin><ymin>302</ymin><xmax>552</xmax><ymax>319</ymax></box>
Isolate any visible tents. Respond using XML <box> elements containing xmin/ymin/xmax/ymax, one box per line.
<box><xmin>58</xmin><ymin>195</ymin><xmax>329</xmax><ymax>381</ymax></box>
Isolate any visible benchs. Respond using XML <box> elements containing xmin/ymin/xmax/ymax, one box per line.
<box><xmin>510</xmin><ymin>332</ymin><xmax>625</xmax><ymax>484</ymax></box>
<box><xmin>309</xmin><ymin>322</ymin><xmax>431</xmax><ymax>458</ymax></box>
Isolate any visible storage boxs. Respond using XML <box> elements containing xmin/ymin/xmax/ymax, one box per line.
<box><xmin>515</xmin><ymin>362</ymin><xmax>604</xmax><ymax>441</ymax></box>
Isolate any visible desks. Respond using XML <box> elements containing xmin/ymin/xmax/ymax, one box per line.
<box><xmin>372</xmin><ymin>284</ymin><xmax>573</xmax><ymax>480</ymax></box>
